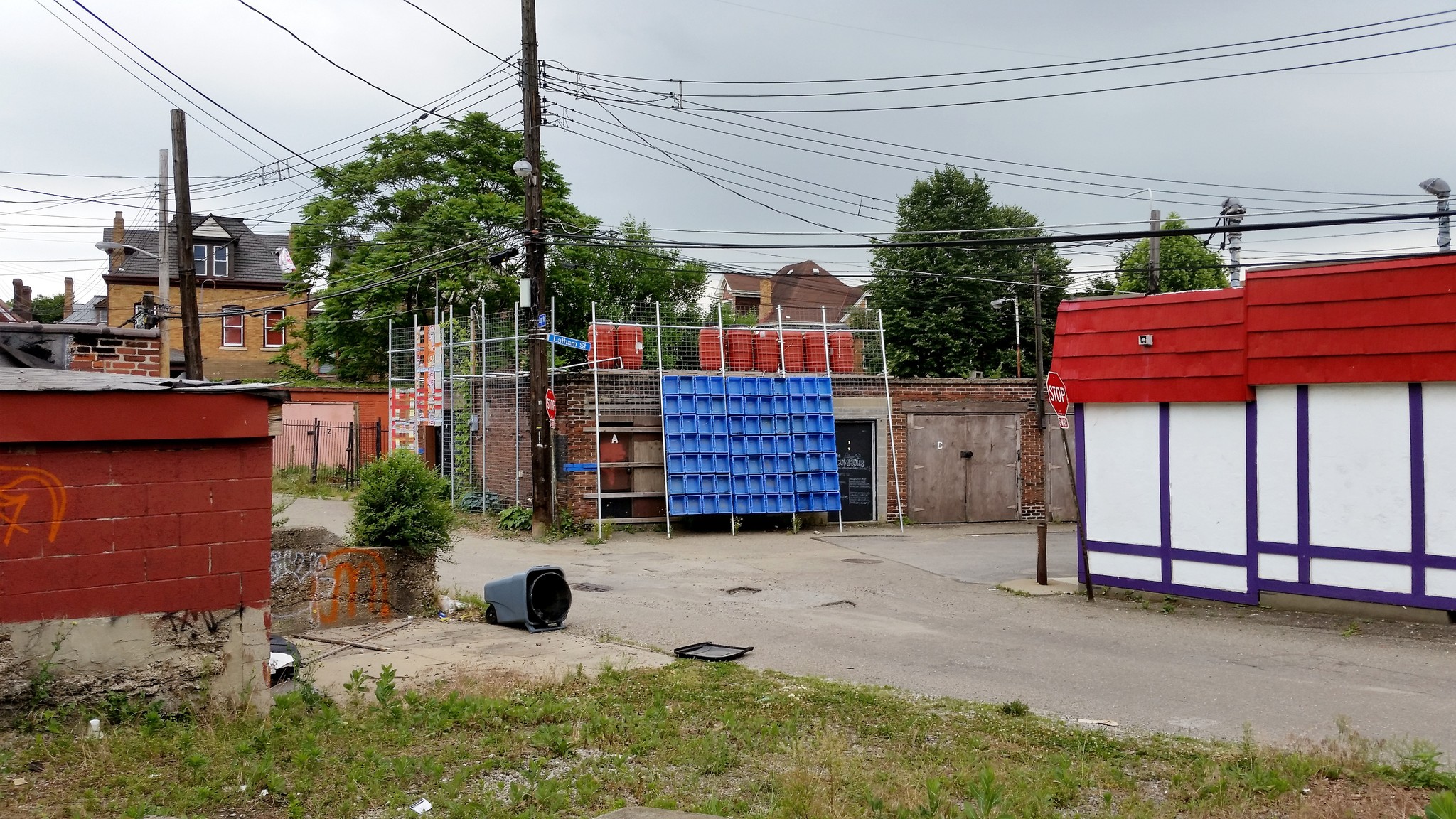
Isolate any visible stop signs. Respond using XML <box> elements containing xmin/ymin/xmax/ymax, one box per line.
<box><xmin>1045</xmin><ymin>371</ymin><xmax>1068</xmax><ymax>417</ymax></box>
<box><xmin>546</xmin><ymin>388</ymin><xmax>556</xmax><ymax>419</ymax></box>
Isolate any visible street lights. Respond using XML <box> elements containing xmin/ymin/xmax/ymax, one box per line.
<box><xmin>95</xmin><ymin>241</ymin><xmax>171</xmax><ymax>380</ymax></box>
<box><xmin>990</xmin><ymin>295</ymin><xmax>1021</xmax><ymax>379</ymax></box>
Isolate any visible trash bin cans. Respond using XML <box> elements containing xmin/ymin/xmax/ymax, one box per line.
<box><xmin>484</xmin><ymin>565</ymin><xmax>572</xmax><ymax>634</ymax></box>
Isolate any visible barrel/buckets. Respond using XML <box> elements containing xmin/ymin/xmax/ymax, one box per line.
<box><xmin>588</xmin><ymin>318</ymin><xmax>616</xmax><ymax>368</ymax></box>
<box><xmin>753</xmin><ymin>324</ymin><xmax>782</xmax><ymax>372</ymax></box>
<box><xmin>699</xmin><ymin>321</ymin><xmax>727</xmax><ymax>371</ymax></box>
<box><xmin>727</xmin><ymin>324</ymin><xmax>752</xmax><ymax>370</ymax></box>
<box><xmin>827</xmin><ymin>326</ymin><xmax>855</xmax><ymax>374</ymax></box>
<box><xmin>781</xmin><ymin>326</ymin><xmax>804</xmax><ymax>373</ymax></box>
<box><xmin>802</xmin><ymin>326</ymin><xmax>829</xmax><ymax>372</ymax></box>
<box><xmin>617</xmin><ymin>320</ymin><xmax>643</xmax><ymax>369</ymax></box>
